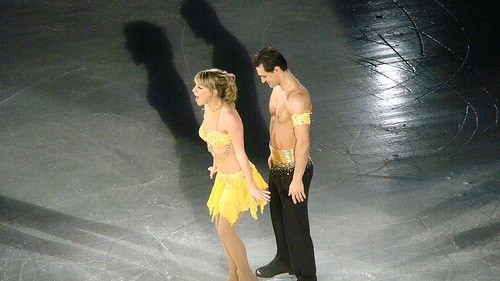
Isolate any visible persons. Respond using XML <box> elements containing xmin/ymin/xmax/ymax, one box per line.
<box><xmin>187</xmin><ymin>68</ymin><xmax>272</xmax><ymax>280</ymax></box>
<box><xmin>250</xmin><ymin>45</ymin><xmax>319</xmax><ymax>281</ymax></box>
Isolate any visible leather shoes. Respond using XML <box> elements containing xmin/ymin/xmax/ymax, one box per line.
<box><xmin>256</xmin><ymin>254</ymin><xmax>295</xmax><ymax>278</ymax></box>
